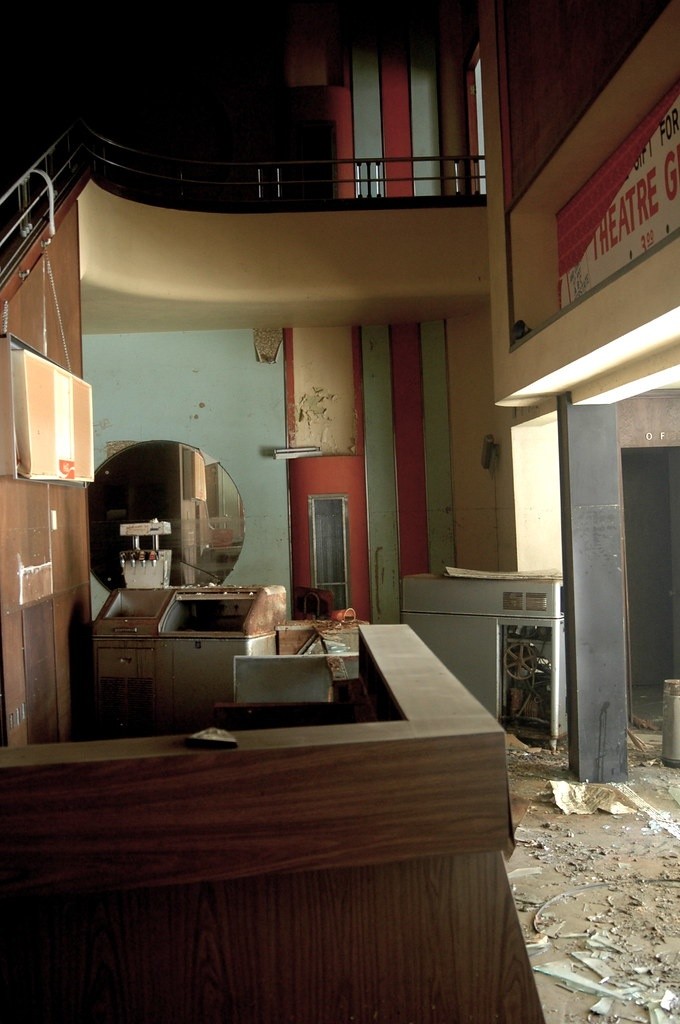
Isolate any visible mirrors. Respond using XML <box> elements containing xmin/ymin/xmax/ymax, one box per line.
<box><xmin>88</xmin><ymin>439</ymin><xmax>244</xmax><ymax>593</ymax></box>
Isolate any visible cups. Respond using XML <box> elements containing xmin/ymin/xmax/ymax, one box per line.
<box><xmin>331</xmin><ymin>608</ymin><xmax>356</xmax><ymax>623</ymax></box>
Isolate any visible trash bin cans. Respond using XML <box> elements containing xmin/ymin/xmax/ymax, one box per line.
<box><xmin>662</xmin><ymin>678</ymin><xmax>680</xmax><ymax>767</ymax></box>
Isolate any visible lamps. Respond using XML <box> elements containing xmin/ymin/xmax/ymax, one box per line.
<box><xmin>273</xmin><ymin>447</ymin><xmax>322</xmax><ymax>459</ymax></box>
